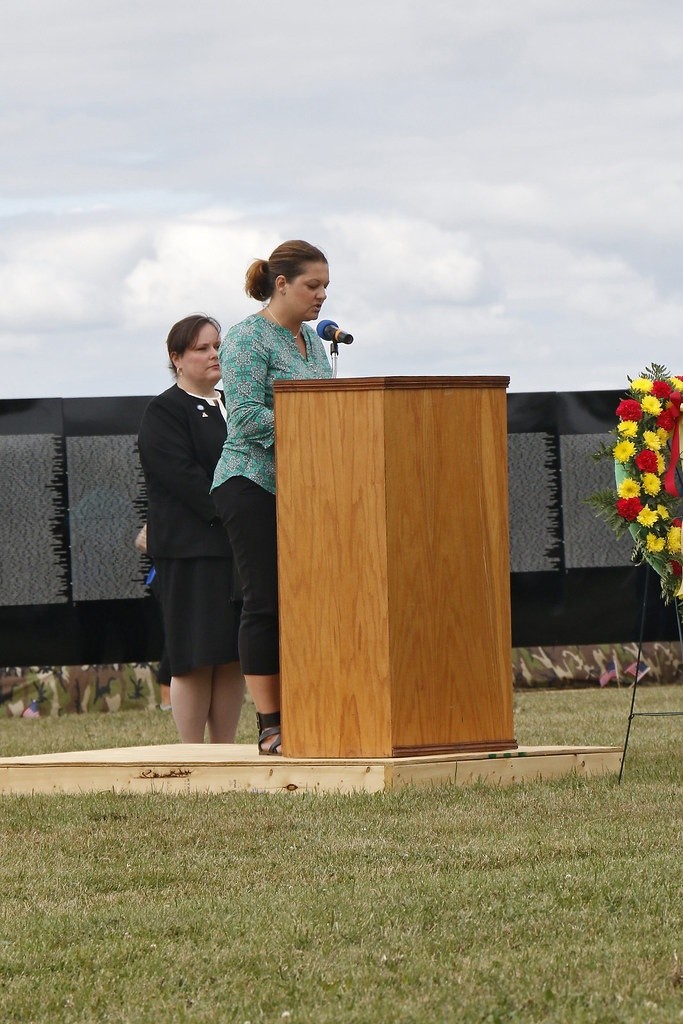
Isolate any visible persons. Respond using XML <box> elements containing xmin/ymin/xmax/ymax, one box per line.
<box><xmin>138</xmin><ymin>315</ymin><xmax>246</xmax><ymax>745</ymax></box>
<box><xmin>211</xmin><ymin>241</ymin><xmax>337</xmax><ymax>757</ymax></box>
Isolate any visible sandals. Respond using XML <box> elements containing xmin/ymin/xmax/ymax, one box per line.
<box><xmin>256</xmin><ymin>712</ymin><xmax>282</xmax><ymax>756</ymax></box>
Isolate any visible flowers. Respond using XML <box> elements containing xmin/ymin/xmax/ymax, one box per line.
<box><xmin>616</xmin><ymin>377</ymin><xmax>683</xmax><ymax>598</ymax></box>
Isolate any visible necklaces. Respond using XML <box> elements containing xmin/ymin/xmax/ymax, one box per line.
<box><xmin>266</xmin><ymin>306</ymin><xmax>300</xmax><ymax>340</ymax></box>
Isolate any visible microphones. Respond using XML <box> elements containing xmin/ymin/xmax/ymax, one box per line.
<box><xmin>316</xmin><ymin>319</ymin><xmax>354</xmax><ymax>345</ymax></box>
